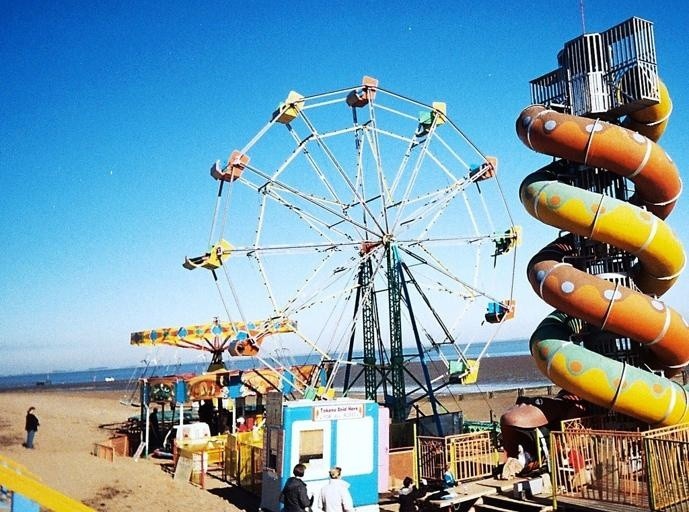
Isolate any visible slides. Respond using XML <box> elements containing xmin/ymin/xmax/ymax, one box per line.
<box><xmin>501</xmin><ymin>66</ymin><xmax>689</xmax><ymax>472</ymax></box>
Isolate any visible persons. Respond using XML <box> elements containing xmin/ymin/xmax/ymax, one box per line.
<box><xmin>496</xmin><ymin>444</ymin><xmax>534</xmax><ymax>480</ymax></box>
<box><xmin>562</xmin><ymin>443</ymin><xmax>590</xmax><ymax>491</ymax></box>
<box><xmin>150</xmin><ymin>399</ymin><xmax>232</xmax><ymax>442</ymax></box>
<box><xmin>441</xmin><ymin>463</ymin><xmax>461</xmax><ymax>491</ymax></box>
<box><xmin>25</xmin><ymin>407</ymin><xmax>39</xmax><ymax>448</ymax></box>
<box><xmin>318</xmin><ymin>467</ymin><xmax>353</xmax><ymax>512</ymax></box>
<box><xmin>188</xmin><ymin>245</ymin><xmax>214</xmax><ymax>264</ymax></box>
<box><xmin>283</xmin><ymin>464</ymin><xmax>314</xmax><ymax>512</ymax></box>
<box><xmin>398</xmin><ymin>477</ymin><xmax>428</xmax><ymax>512</ymax></box>
<box><xmin>495</xmin><ymin>238</ymin><xmax>510</xmax><ymax>254</ymax></box>
